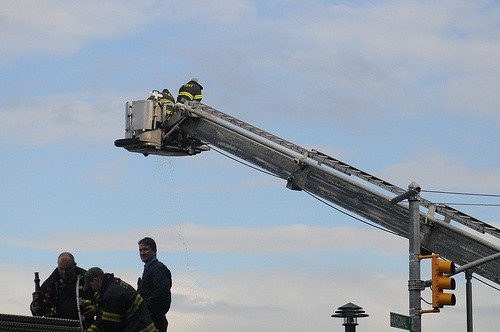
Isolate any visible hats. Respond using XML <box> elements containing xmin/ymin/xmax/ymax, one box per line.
<box><xmin>82</xmin><ymin>267</ymin><xmax>104</xmax><ymax>291</ymax></box>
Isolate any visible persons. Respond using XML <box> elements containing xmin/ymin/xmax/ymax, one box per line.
<box><xmin>29</xmin><ymin>252</ymin><xmax>99</xmax><ymax>330</ymax></box>
<box><xmin>147</xmin><ymin>77</ymin><xmax>204</xmax><ymax>147</ymax></box>
<box><xmin>137</xmin><ymin>237</ymin><xmax>172</xmax><ymax>332</ymax></box>
<box><xmin>82</xmin><ymin>267</ymin><xmax>159</xmax><ymax>332</ymax></box>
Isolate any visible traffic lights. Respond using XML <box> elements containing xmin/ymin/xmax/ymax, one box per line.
<box><xmin>432</xmin><ymin>254</ymin><xmax>456</xmax><ymax>311</ymax></box>
